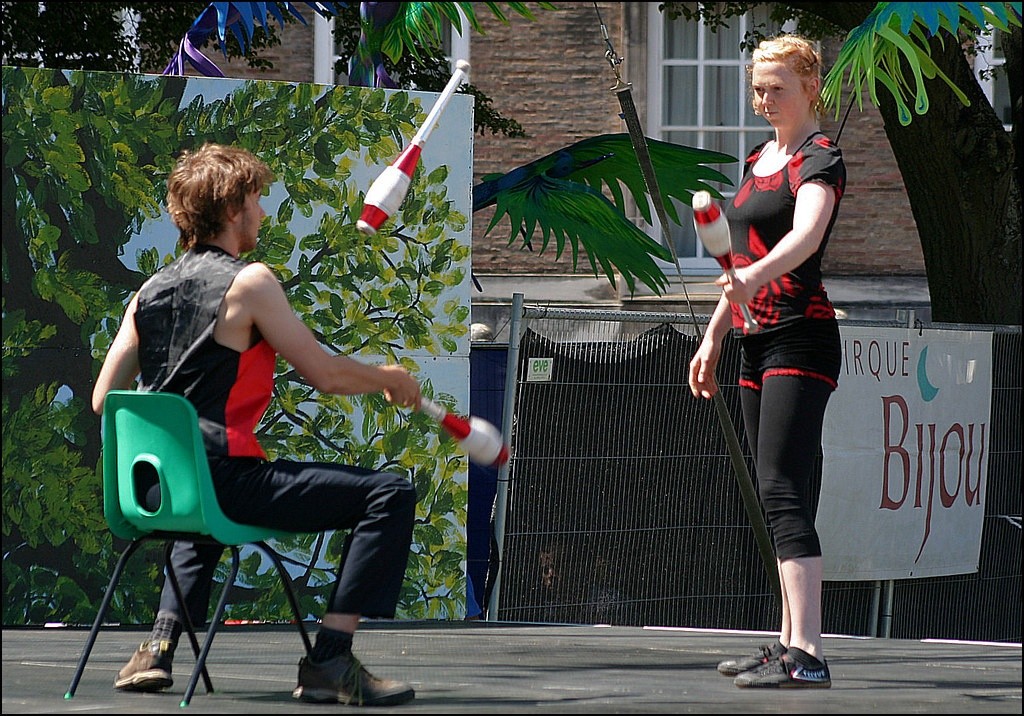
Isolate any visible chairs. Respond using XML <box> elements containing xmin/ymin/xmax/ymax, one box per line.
<box><xmin>63</xmin><ymin>390</ymin><xmax>313</xmax><ymax>707</ymax></box>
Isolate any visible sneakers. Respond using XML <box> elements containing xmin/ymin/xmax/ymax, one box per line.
<box><xmin>717</xmin><ymin>643</ymin><xmax>783</xmax><ymax>677</ymax></box>
<box><xmin>733</xmin><ymin>653</ymin><xmax>832</xmax><ymax>689</ymax></box>
<box><xmin>113</xmin><ymin>639</ymin><xmax>175</xmax><ymax>691</ymax></box>
<box><xmin>293</xmin><ymin>650</ymin><xmax>415</xmax><ymax>708</ymax></box>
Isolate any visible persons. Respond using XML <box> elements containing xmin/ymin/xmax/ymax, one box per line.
<box><xmin>92</xmin><ymin>145</ymin><xmax>420</xmax><ymax>705</ymax></box>
<box><xmin>690</xmin><ymin>35</ymin><xmax>846</xmax><ymax>688</ymax></box>
